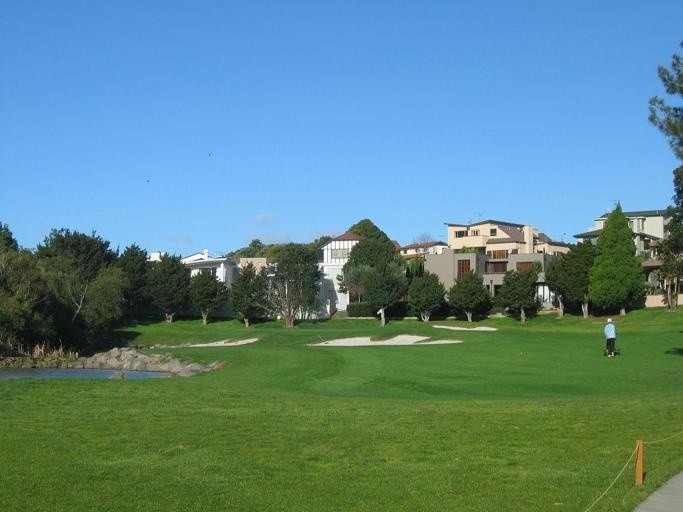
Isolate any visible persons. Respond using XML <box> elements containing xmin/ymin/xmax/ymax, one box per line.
<box><xmin>603</xmin><ymin>318</ymin><xmax>616</xmax><ymax>357</ymax></box>
